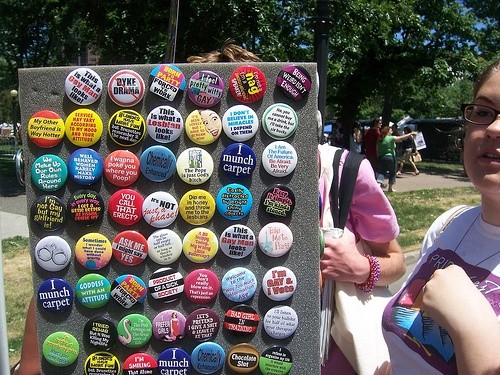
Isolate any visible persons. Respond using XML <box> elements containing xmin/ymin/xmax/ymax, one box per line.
<box><xmin>15</xmin><ymin>44</ymin><xmax>423</xmax><ymax>375</ymax></box>
<box><xmin>375</xmin><ymin>59</ymin><xmax>500</xmax><ymax>375</ymax></box>
<box><xmin>375</xmin><ymin>126</ymin><xmax>417</xmax><ymax>192</ymax></box>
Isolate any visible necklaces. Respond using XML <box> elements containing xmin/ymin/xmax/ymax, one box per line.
<box><xmin>445</xmin><ymin>213</ymin><xmax>500</xmax><ymax>274</ymax></box>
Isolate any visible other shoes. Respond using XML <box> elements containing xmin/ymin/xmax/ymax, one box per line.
<box><xmin>388</xmin><ymin>188</ymin><xmax>397</xmax><ymax>192</ymax></box>
<box><xmin>396</xmin><ymin>171</ymin><xmax>401</xmax><ymax>175</ymax></box>
<box><xmin>415</xmin><ymin>170</ymin><xmax>419</xmax><ymax>175</ymax></box>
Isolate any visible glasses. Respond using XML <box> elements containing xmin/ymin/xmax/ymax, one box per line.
<box><xmin>460</xmin><ymin>103</ymin><xmax>500</xmax><ymax>125</ymax></box>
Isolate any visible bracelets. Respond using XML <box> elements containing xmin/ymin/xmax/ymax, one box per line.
<box><xmin>355</xmin><ymin>253</ymin><xmax>376</xmax><ymax>289</ymax></box>
<box><xmin>362</xmin><ymin>255</ymin><xmax>380</xmax><ymax>293</ymax></box>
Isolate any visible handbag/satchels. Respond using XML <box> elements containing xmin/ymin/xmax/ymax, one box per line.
<box><xmin>332</xmin><ymin>240</ymin><xmax>394</xmax><ymax>375</ymax></box>
<box><xmin>413</xmin><ymin>152</ymin><xmax>422</xmax><ymax>162</ymax></box>
